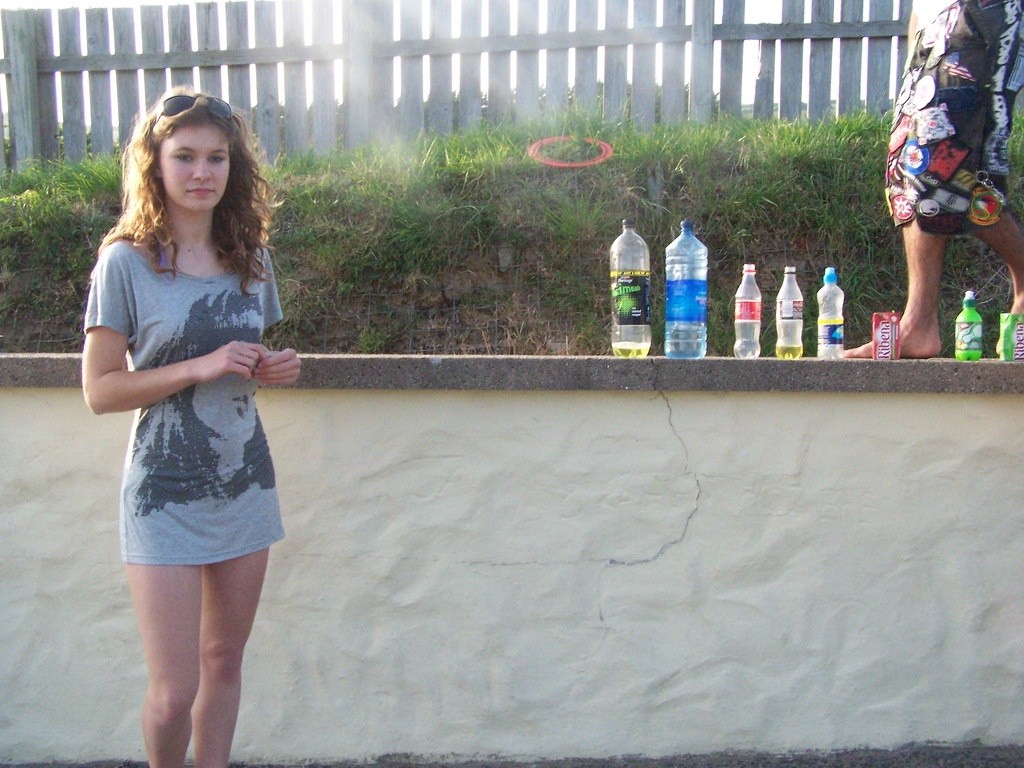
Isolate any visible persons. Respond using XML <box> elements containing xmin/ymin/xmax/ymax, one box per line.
<box><xmin>837</xmin><ymin>1</ymin><xmax>1024</xmax><ymax>359</ymax></box>
<box><xmin>75</xmin><ymin>87</ymin><xmax>305</xmax><ymax>768</ymax></box>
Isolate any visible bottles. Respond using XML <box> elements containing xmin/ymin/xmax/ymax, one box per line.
<box><xmin>610</xmin><ymin>218</ymin><xmax>654</xmax><ymax>358</ymax></box>
<box><xmin>775</xmin><ymin>266</ymin><xmax>805</xmax><ymax>359</ymax></box>
<box><xmin>732</xmin><ymin>263</ymin><xmax>763</xmax><ymax>359</ymax></box>
<box><xmin>664</xmin><ymin>221</ymin><xmax>710</xmax><ymax>358</ymax></box>
<box><xmin>816</xmin><ymin>266</ymin><xmax>845</xmax><ymax>359</ymax></box>
<box><xmin>955</xmin><ymin>291</ymin><xmax>983</xmax><ymax>361</ymax></box>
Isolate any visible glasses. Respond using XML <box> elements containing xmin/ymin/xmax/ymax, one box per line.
<box><xmin>150</xmin><ymin>93</ymin><xmax>233</xmax><ymax>136</ymax></box>
